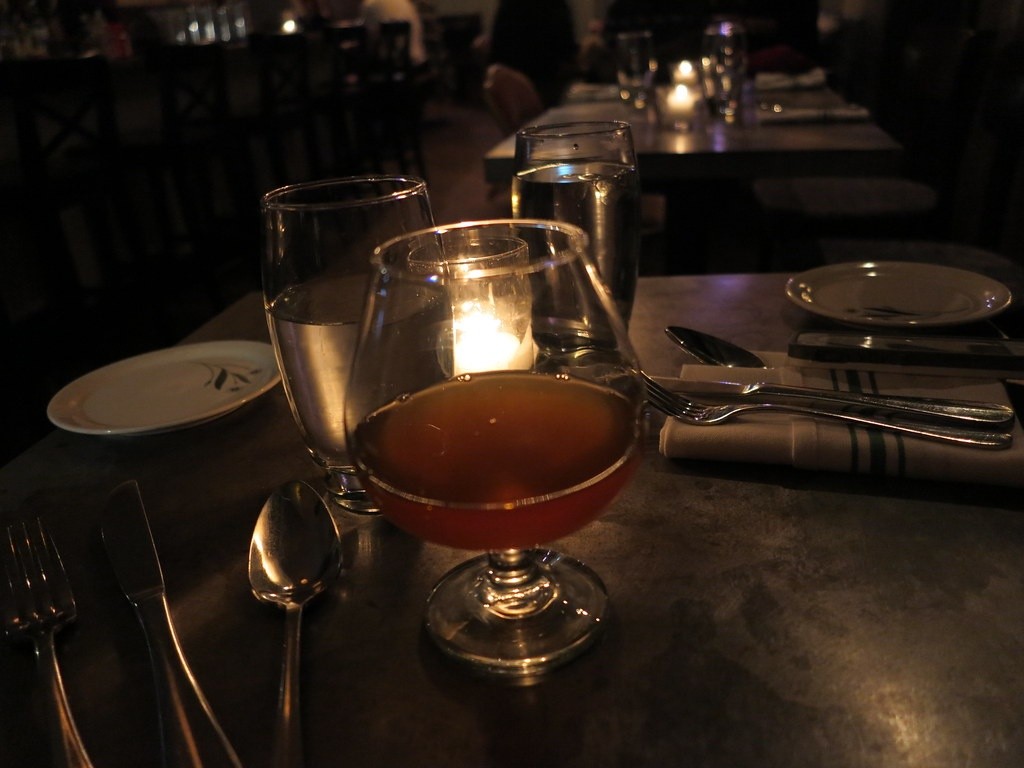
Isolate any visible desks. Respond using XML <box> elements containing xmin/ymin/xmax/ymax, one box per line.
<box><xmin>0</xmin><ymin>271</ymin><xmax>1024</xmax><ymax>768</ymax></box>
<box><xmin>483</xmin><ymin>83</ymin><xmax>907</xmax><ymax>275</ymax></box>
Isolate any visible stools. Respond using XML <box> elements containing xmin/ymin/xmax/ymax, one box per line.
<box><xmin>0</xmin><ymin>23</ymin><xmax>423</xmax><ymax>385</ymax></box>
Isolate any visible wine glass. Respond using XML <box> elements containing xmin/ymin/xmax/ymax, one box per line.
<box><xmin>347</xmin><ymin>216</ymin><xmax>646</xmax><ymax>688</ymax></box>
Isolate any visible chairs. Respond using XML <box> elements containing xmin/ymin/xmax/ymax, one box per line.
<box><xmin>480</xmin><ymin>63</ymin><xmax>666</xmax><ymax>259</ymax></box>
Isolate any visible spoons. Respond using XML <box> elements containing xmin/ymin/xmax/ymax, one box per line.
<box><xmin>664</xmin><ymin>325</ymin><xmax>1013</xmax><ymax>428</ymax></box>
<box><xmin>245</xmin><ymin>479</ymin><xmax>343</xmax><ymax>768</ymax></box>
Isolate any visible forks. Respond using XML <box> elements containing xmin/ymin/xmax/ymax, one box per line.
<box><xmin>634</xmin><ymin>371</ymin><xmax>1013</xmax><ymax>450</ymax></box>
<box><xmin>1</xmin><ymin>517</ymin><xmax>99</xmax><ymax>768</ymax></box>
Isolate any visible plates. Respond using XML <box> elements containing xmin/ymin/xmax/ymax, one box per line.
<box><xmin>46</xmin><ymin>340</ymin><xmax>283</xmax><ymax>437</ymax></box>
<box><xmin>786</xmin><ymin>260</ymin><xmax>1014</xmax><ymax>333</ymax></box>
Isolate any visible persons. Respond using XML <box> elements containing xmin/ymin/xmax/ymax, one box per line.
<box><xmin>361</xmin><ymin>1</ymin><xmax>424</xmax><ymax>142</ymax></box>
<box><xmin>487</xmin><ymin>1</ymin><xmax>576</xmax><ymax>112</ymax></box>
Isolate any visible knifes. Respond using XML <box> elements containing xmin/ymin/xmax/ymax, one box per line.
<box><xmin>656</xmin><ymin>380</ymin><xmax>1015</xmax><ymax>428</ymax></box>
<box><xmin>100</xmin><ymin>478</ymin><xmax>211</xmax><ymax>768</ymax></box>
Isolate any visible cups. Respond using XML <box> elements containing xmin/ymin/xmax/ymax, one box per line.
<box><xmin>615</xmin><ymin>14</ymin><xmax>749</xmax><ymax>127</ymax></box>
<box><xmin>260</xmin><ymin>173</ymin><xmax>440</xmax><ymax>516</ymax></box>
<box><xmin>408</xmin><ymin>119</ymin><xmax>641</xmax><ymax>395</ymax></box>
